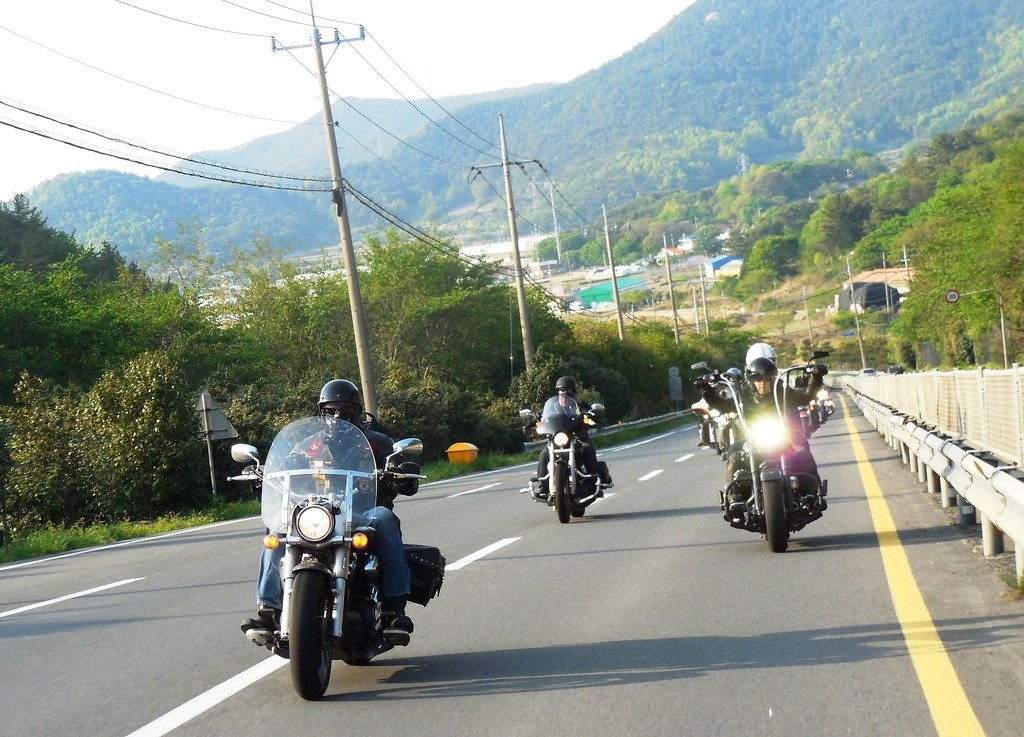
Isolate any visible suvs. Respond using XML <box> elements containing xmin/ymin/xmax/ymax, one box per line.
<box><xmin>887</xmin><ymin>366</ymin><xmax>904</xmax><ymax>374</ymax></box>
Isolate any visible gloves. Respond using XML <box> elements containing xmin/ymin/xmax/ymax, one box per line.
<box><xmin>589</xmin><ymin>412</ymin><xmax>600</xmax><ymax>423</ymax></box>
<box><xmin>813</xmin><ymin>361</ymin><xmax>829</xmax><ymax>376</ymax></box>
<box><xmin>399</xmin><ymin>461</ymin><xmax>420</xmax><ymax>475</ymax></box>
<box><xmin>524</xmin><ymin>418</ymin><xmax>534</xmax><ymax>429</ymax></box>
<box><xmin>241</xmin><ymin>465</ymin><xmax>261</xmax><ymax>475</ymax></box>
<box><xmin>694</xmin><ymin>375</ymin><xmax>707</xmax><ymax>389</ymax></box>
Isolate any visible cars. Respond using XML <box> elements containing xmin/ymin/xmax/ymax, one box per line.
<box><xmin>861</xmin><ymin>368</ymin><xmax>876</xmax><ymax>377</ymax></box>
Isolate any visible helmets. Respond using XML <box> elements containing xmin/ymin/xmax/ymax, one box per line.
<box><xmin>728</xmin><ymin>368</ymin><xmax>742</xmax><ymax>377</ymax></box>
<box><xmin>317</xmin><ymin>379</ymin><xmax>364</xmax><ymax>407</ymax></box>
<box><xmin>556</xmin><ymin>376</ymin><xmax>580</xmax><ymax>391</ymax></box>
<box><xmin>746</xmin><ymin>343</ymin><xmax>781</xmax><ymax>364</ymax></box>
<box><xmin>750</xmin><ymin>358</ymin><xmax>781</xmax><ymax>378</ymax></box>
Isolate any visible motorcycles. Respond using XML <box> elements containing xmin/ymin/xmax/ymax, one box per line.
<box><xmin>227</xmin><ymin>437</ymin><xmax>446</xmax><ymax>700</ymax></box>
<box><xmin>519</xmin><ymin>395</ymin><xmax>612</xmax><ymax>523</ymax></box>
<box><xmin>691</xmin><ymin>350</ymin><xmax>835</xmax><ymax>553</ymax></box>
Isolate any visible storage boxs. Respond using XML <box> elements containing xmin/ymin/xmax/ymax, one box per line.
<box><xmin>373</xmin><ymin>543</ymin><xmax>446</xmax><ymax>607</ymax></box>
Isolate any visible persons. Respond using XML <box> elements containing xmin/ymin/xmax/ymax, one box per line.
<box><xmin>241</xmin><ymin>380</ymin><xmax>414</xmax><ymax>635</ymax></box>
<box><xmin>694</xmin><ymin>342</ymin><xmax>827</xmax><ymax>522</ymax></box>
<box><xmin>525</xmin><ymin>376</ymin><xmax>607</xmax><ymax>493</ymax></box>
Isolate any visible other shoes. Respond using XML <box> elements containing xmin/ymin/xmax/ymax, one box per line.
<box><xmin>697</xmin><ymin>440</ymin><xmax>708</xmax><ymax>448</ymax></box>
<box><xmin>602</xmin><ymin>474</ymin><xmax>615</xmax><ymax>488</ymax></box>
<box><xmin>240</xmin><ymin>606</ymin><xmax>278</xmax><ymax>633</ymax></box>
<box><xmin>383</xmin><ymin>612</ymin><xmax>415</xmax><ymax>635</ymax></box>
<box><xmin>721</xmin><ymin>452</ymin><xmax>726</xmax><ymax>461</ymax></box>
<box><xmin>537</xmin><ymin>486</ymin><xmax>550</xmax><ymax>499</ymax></box>
<box><xmin>731</xmin><ymin>509</ymin><xmax>746</xmax><ymax>526</ymax></box>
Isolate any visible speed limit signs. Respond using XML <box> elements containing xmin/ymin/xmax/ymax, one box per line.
<box><xmin>946</xmin><ymin>290</ymin><xmax>960</xmax><ymax>303</ymax></box>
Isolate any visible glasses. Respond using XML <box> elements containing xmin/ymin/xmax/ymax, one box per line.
<box><xmin>322</xmin><ymin>407</ymin><xmax>360</xmax><ymax>417</ymax></box>
